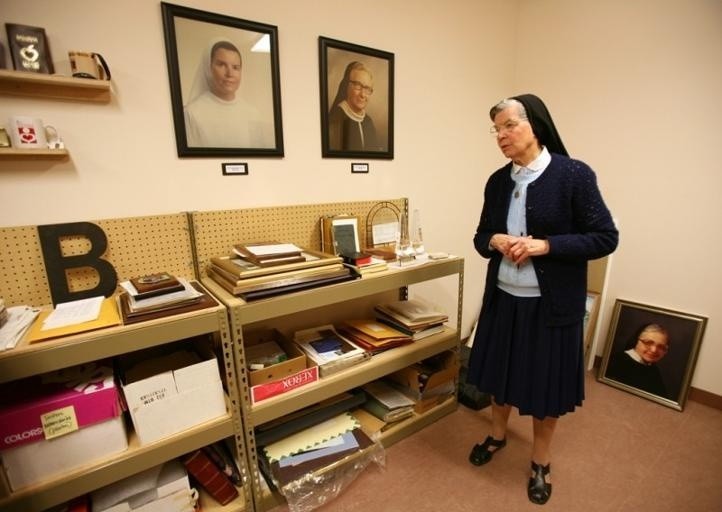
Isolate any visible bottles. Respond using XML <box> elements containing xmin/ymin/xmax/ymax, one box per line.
<box><xmin>394</xmin><ymin>207</ymin><xmax>412</xmax><ymax>253</ymax></box>
<box><xmin>411</xmin><ymin>210</ymin><xmax>425</xmax><ymax>256</ymax></box>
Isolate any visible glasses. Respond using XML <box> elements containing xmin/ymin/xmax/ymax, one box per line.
<box><xmin>350</xmin><ymin>79</ymin><xmax>373</xmax><ymax>95</ymax></box>
<box><xmin>489</xmin><ymin>119</ymin><xmax>517</xmax><ymax>133</ymax></box>
<box><xmin>638</xmin><ymin>338</ymin><xmax>668</xmax><ymax>351</ymax></box>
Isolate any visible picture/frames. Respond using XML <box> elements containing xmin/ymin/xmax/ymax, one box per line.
<box><xmin>159</xmin><ymin>0</ymin><xmax>286</xmax><ymax>163</ymax></box>
<box><xmin>582</xmin><ymin>289</ymin><xmax>601</xmax><ymax>356</ymax></box>
<box><xmin>595</xmin><ymin>298</ymin><xmax>710</xmax><ymax>414</ymax></box>
<box><xmin>316</xmin><ymin>33</ymin><xmax>397</xmax><ymax>163</ymax></box>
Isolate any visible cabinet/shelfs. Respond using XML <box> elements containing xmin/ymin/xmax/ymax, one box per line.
<box><xmin>1</xmin><ymin>254</ymin><xmax>252</xmax><ymax>511</ymax></box>
<box><xmin>0</xmin><ymin>68</ymin><xmax>115</xmax><ymax>166</ymax></box>
<box><xmin>203</xmin><ymin>246</ymin><xmax>467</xmax><ymax>509</ymax></box>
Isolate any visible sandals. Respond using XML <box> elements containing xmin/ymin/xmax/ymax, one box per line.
<box><xmin>469</xmin><ymin>435</ymin><xmax>506</xmax><ymax>466</ymax></box>
<box><xmin>527</xmin><ymin>461</ymin><xmax>551</xmax><ymax>504</ymax></box>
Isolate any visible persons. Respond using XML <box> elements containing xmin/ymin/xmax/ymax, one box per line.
<box><xmin>328</xmin><ymin>61</ymin><xmax>377</xmax><ymax>151</ymax></box>
<box><xmin>183</xmin><ymin>41</ymin><xmax>270</xmax><ymax>148</ymax></box>
<box><xmin>607</xmin><ymin>322</ymin><xmax>669</xmax><ymax>396</ymax></box>
<box><xmin>463</xmin><ymin>92</ymin><xmax>620</xmax><ymax>504</ymax></box>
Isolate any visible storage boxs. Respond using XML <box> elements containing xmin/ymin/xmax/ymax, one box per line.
<box><xmin>242</xmin><ymin>328</ymin><xmax>307</xmax><ymax>389</ymax></box>
<box><xmin>0</xmin><ymin>353</ymin><xmax>132</xmax><ymax>493</ymax></box>
<box><xmin>120</xmin><ymin>335</ymin><xmax>229</xmax><ymax>452</ymax></box>
<box><xmin>396</xmin><ymin>366</ymin><xmax>456</xmax><ymax>416</ymax></box>
<box><xmin>245</xmin><ymin>350</ymin><xmax>321</xmax><ymax>405</ymax></box>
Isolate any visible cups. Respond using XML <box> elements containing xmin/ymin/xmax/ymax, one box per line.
<box><xmin>68</xmin><ymin>49</ymin><xmax>111</xmax><ymax>80</ymax></box>
<box><xmin>11</xmin><ymin>116</ymin><xmax>61</xmax><ymax>150</ymax></box>
